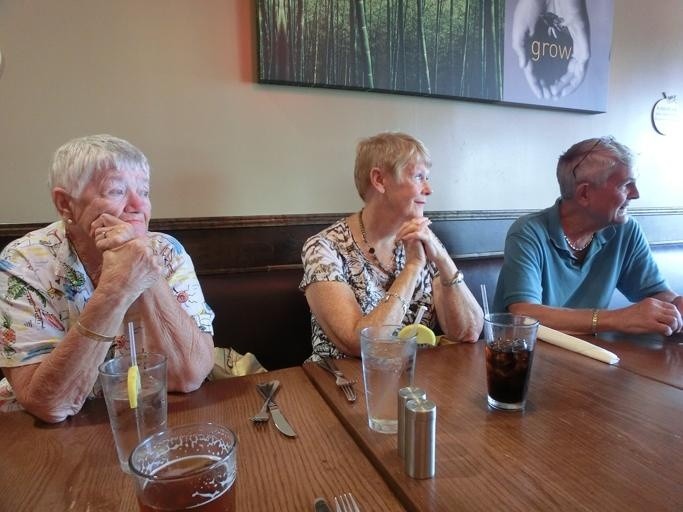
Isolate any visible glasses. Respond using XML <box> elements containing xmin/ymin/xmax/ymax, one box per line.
<box><xmin>573</xmin><ymin>134</ymin><xmax>617</xmax><ymax>178</ymax></box>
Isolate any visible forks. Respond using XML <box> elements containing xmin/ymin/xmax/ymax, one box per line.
<box><xmin>247</xmin><ymin>380</ymin><xmax>280</xmax><ymax>422</ymax></box>
<box><xmin>334</xmin><ymin>492</ymin><xmax>361</xmax><ymax>512</ymax></box>
<box><xmin>316</xmin><ymin>360</ymin><xmax>357</xmax><ymax>387</ymax></box>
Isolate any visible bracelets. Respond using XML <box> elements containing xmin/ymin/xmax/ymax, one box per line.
<box><xmin>382</xmin><ymin>288</ymin><xmax>410</xmax><ymax>319</ymax></box>
<box><xmin>586</xmin><ymin>306</ymin><xmax>601</xmax><ymax>339</ymax></box>
<box><xmin>69</xmin><ymin>317</ymin><xmax>119</xmax><ymax>351</ymax></box>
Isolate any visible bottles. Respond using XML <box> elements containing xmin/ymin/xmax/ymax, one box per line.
<box><xmin>397</xmin><ymin>385</ymin><xmax>427</xmax><ymax>460</ymax></box>
<box><xmin>404</xmin><ymin>398</ymin><xmax>437</xmax><ymax>479</ymax></box>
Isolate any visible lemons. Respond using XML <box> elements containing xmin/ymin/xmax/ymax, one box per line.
<box><xmin>127</xmin><ymin>365</ymin><xmax>141</xmax><ymax>410</ymax></box>
<box><xmin>399</xmin><ymin>324</ymin><xmax>436</xmax><ymax>345</ymax></box>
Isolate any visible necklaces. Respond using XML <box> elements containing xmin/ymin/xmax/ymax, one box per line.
<box><xmin>557</xmin><ymin>230</ymin><xmax>595</xmax><ymax>253</ymax></box>
<box><xmin>354</xmin><ymin>206</ymin><xmax>403</xmax><ymax>280</ymax></box>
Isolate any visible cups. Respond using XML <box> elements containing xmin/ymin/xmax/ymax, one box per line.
<box><xmin>99</xmin><ymin>351</ymin><xmax>169</xmax><ymax>474</ymax></box>
<box><xmin>360</xmin><ymin>324</ymin><xmax>419</xmax><ymax>435</ymax></box>
<box><xmin>129</xmin><ymin>423</ymin><xmax>238</xmax><ymax>512</ymax></box>
<box><xmin>483</xmin><ymin>312</ymin><xmax>539</xmax><ymax>414</ymax></box>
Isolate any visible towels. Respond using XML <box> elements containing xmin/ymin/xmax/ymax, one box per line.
<box><xmin>524</xmin><ymin>317</ymin><xmax>620</xmax><ymax>365</ymax></box>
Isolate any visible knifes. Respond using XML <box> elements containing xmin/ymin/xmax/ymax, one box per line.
<box><xmin>254</xmin><ymin>384</ymin><xmax>296</xmax><ymax>437</ymax></box>
<box><xmin>321</xmin><ymin>354</ymin><xmax>357</xmax><ymax>402</ymax></box>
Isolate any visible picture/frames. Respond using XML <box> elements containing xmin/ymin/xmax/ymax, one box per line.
<box><xmin>253</xmin><ymin>0</ymin><xmax>613</xmax><ymax>113</ymax></box>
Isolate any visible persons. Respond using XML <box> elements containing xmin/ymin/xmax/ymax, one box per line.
<box><xmin>490</xmin><ymin>137</ymin><xmax>683</xmax><ymax>337</ymax></box>
<box><xmin>297</xmin><ymin>129</ymin><xmax>485</xmax><ymax>364</ymax></box>
<box><xmin>0</xmin><ymin>134</ymin><xmax>216</xmax><ymax>424</ymax></box>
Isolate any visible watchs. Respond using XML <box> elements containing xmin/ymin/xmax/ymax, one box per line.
<box><xmin>437</xmin><ymin>270</ymin><xmax>466</xmax><ymax>293</ymax></box>
